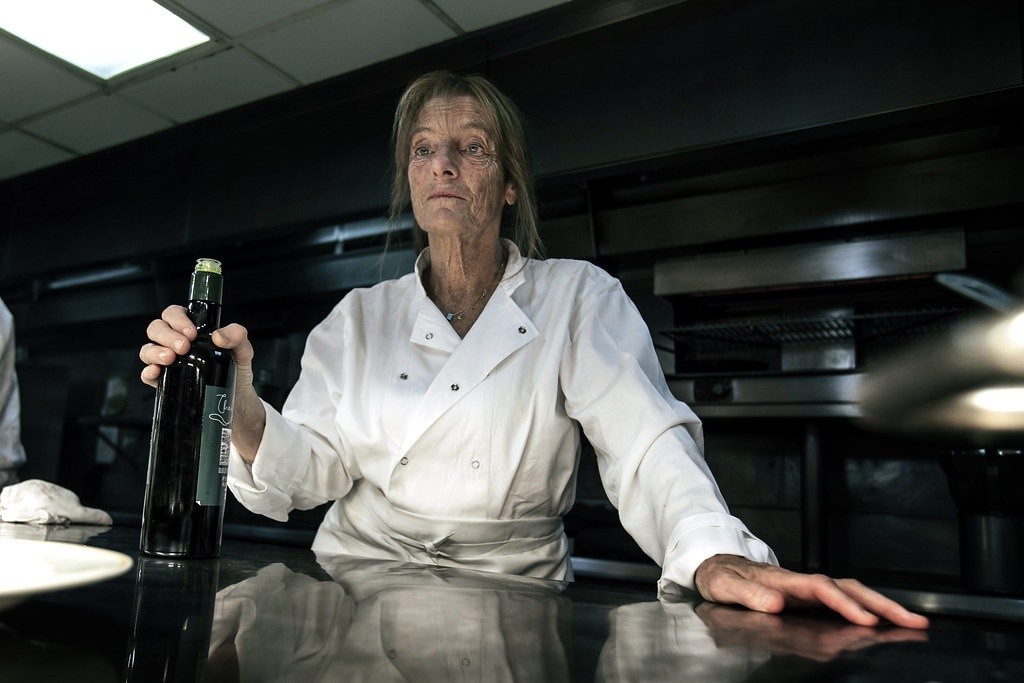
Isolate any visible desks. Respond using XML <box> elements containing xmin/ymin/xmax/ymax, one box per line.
<box><xmin>0</xmin><ymin>526</ymin><xmax>1024</xmax><ymax>683</ymax></box>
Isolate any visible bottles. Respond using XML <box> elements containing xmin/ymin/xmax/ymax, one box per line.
<box><xmin>137</xmin><ymin>257</ymin><xmax>238</xmax><ymax>561</ymax></box>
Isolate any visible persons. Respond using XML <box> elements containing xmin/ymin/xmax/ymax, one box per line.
<box><xmin>139</xmin><ymin>70</ymin><xmax>929</xmax><ymax>630</ymax></box>
<box><xmin>215</xmin><ymin>562</ymin><xmax>928</xmax><ymax>683</ymax></box>
<box><xmin>0</xmin><ymin>291</ymin><xmax>25</xmax><ymax>491</ymax></box>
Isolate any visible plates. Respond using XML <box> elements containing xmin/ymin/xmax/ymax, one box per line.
<box><xmin>0</xmin><ymin>539</ymin><xmax>134</xmax><ymax>621</ymax></box>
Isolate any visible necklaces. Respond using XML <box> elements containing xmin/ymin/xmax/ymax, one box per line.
<box><xmin>427</xmin><ymin>259</ymin><xmax>503</xmax><ymax>320</ymax></box>
